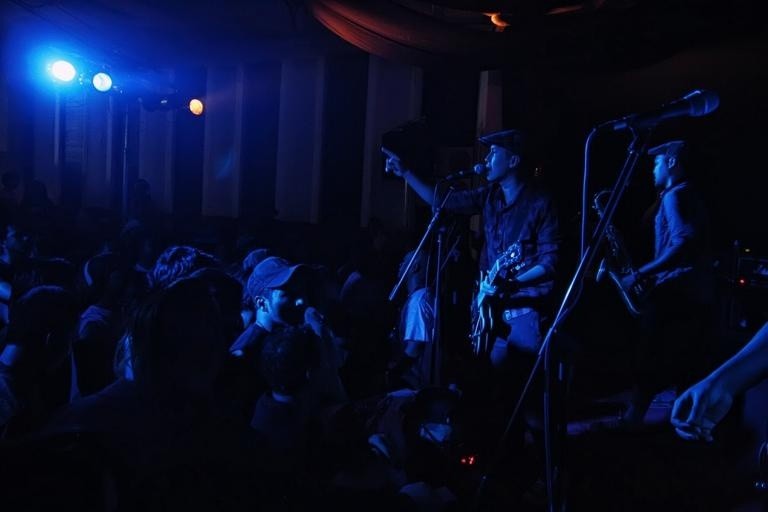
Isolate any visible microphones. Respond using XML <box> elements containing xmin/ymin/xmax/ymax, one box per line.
<box><xmin>601</xmin><ymin>89</ymin><xmax>720</xmax><ymax>133</ymax></box>
<box><xmin>446</xmin><ymin>163</ymin><xmax>485</xmax><ymax>182</ymax></box>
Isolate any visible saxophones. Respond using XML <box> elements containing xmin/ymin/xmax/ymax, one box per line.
<box><xmin>592</xmin><ymin>189</ymin><xmax>649</xmax><ymax>317</ymax></box>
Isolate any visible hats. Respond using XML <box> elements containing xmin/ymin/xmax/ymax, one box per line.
<box><xmin>477</xmin><ymin>129</ymin><xmax>528</xmax><ymax>156</ymax></box>
<box><xmin>247</xmin><ymin>255</ymin><xmax>318</xmax><ymax>298</ymax></box>
<box><xmin>647</xmin><ymin>140</ymin><xmax>703</xmax><ymax>169</ymax></box>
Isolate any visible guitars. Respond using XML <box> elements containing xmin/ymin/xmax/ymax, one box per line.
<box><xmin>468</xmin><ymin>239</ymin><xmax>524</xmax><ymax>357</ymax></box>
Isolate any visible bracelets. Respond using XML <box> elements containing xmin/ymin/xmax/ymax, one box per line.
<box><xmin>631</xmin><ymin>266</ymin><xmax>646</xmax><ymax>286</ymax></box>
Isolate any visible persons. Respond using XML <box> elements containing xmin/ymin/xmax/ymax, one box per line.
<box><xmin>615</xmin><ymin>136</ymin><xmax>722</xmax><ymax>362</ymax></box>
<box><xmin>662</xmin><ymin>318</ymin><xmax>768</xmax><ymax>451</ymax></box>
<box><xmin>377</xmin><ymin>127</ymin><xmax>561</xmax><ymax>414</ymax></box>
